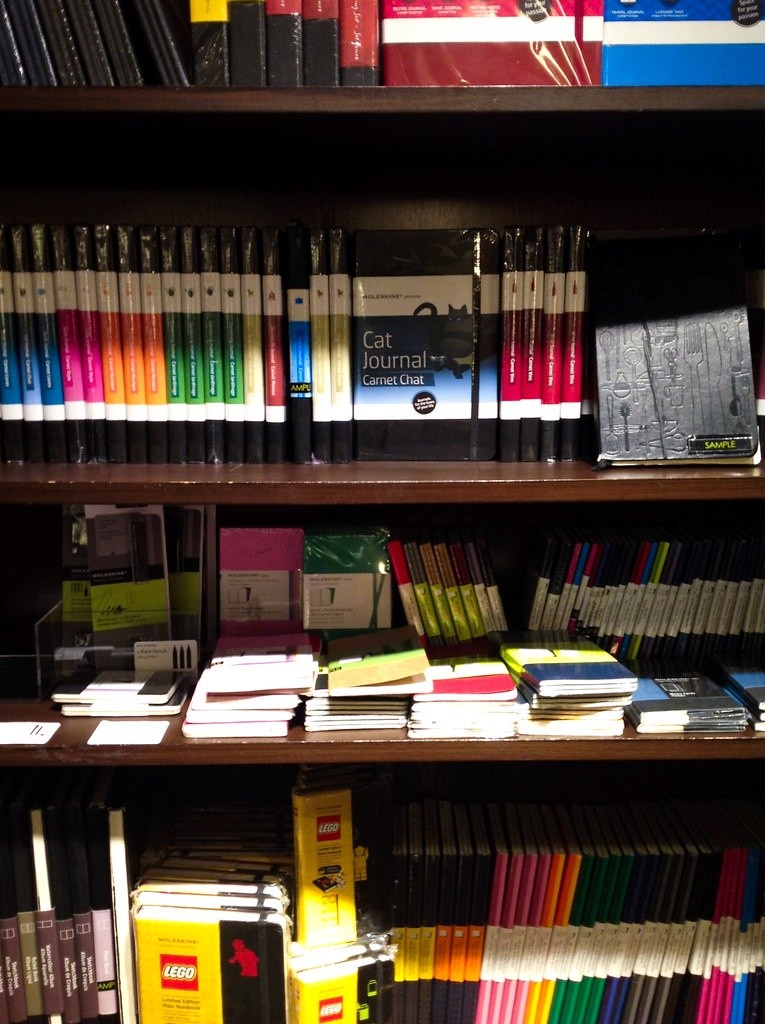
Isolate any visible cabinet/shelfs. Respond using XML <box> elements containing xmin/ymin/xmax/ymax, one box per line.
<box><xmin>1</xmin><ymin>83</ymin><xmax>765</xmax><ymax>769</ymax></box>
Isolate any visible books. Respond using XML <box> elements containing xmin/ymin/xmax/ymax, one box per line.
<box><xmin>0</xmin><ymin>224</ymin><xmax>765</xmax><ymax>470</ymax></box>
<box><xmin>0</xmin><ymin>0</ymin><xmax>765</xmax><ymax>87</ymax></box>
<box><xmin>0</xmin><ymin>779</ymin><xmax>765</xmax><ymax>1024</ymax></box>
<box><xmin>29</xmin><ymin>499</ymin><xmax>765</xmax><ymax>738</ymax></box>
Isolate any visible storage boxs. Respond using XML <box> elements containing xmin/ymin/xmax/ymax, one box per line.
<box><xmin>380</xmin><ymin>0</ymin><xmax>604</xmax><ymax>86</ymax></box>
<box><xmin>602</xmin><ymin>1</ymin><xmax>765</xmax><ymax>86</ymax></box>
